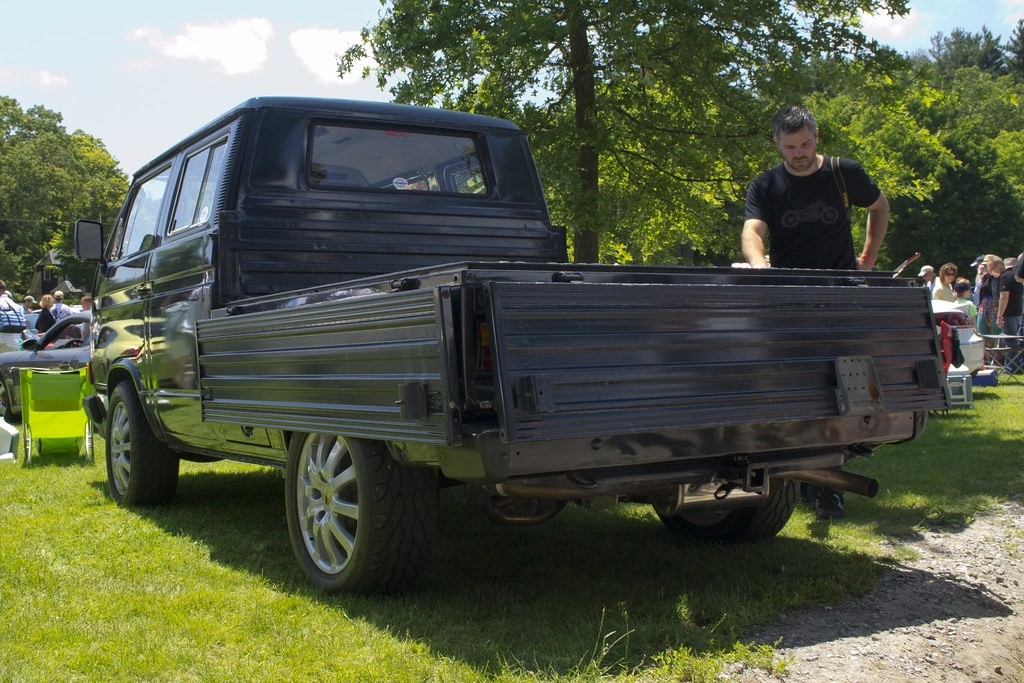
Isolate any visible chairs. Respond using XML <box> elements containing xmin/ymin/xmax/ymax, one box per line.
<box><xmin>19</xmin><ymin>366</ymin><xmax>95</xmax><ymax>464</ymax></box>
<box><xmin>984</xmin><ymin>339</ymin><xmax>1024</xmax><ymax>385</ymax></box>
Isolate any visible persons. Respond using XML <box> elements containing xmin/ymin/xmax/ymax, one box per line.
<box><xmin>918</xmin><ymin>253</ymin><xmax>1024</xmax><ymax>375</ymax></box>
<box><xmin>0</xmin><ymin>280</ymin><xmax>99</xmax><ymax>415</ymax></box>
<box><xmin>742</xmin><ymin>107</ymin><xmax>890</xmax><ymax>268</ymax></box>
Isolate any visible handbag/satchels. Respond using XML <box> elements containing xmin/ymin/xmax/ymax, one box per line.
<box><xmin>0</xmin><ymin>299</ymin><xmax>26</xmax><ymax>333</ymax></box>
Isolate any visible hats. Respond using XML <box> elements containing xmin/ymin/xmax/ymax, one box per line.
<box><xmin>970</xmin><ymin>255</ymin><xmax>984</xmax><ymax>266</ymax></box>
<box><xmin>918</xmin><ymin>265</ymin><xmax>933</xmax><ymax>277</ymax></box>
<box><xmin>54</xmin><ymin>291</ymin><xmax>63</xmax><ymax>298</ymax></box>
<box><xmin>24</xmin><ymin>296</ymin><xmax>37</xmax><ymax>303</ymax></box>
<box><xmin>981</xmin><ymin>255</ymin><xmax>995</xmax><ymax>264</ymax></box>
<box><xmin>954</xmin><ymin>282</ymin><xmax>969</xmax><ymax>292</ymax></box>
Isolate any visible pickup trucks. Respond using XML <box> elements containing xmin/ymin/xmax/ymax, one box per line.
<box><xmin>74</xmin><ymin>96</ymin><xmax>955</xmax><ymax>602</ymax></box>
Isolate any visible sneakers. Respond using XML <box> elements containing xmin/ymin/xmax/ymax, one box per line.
<box><xmin>800</xmin><ymin>483</ymin><xmax>845</xmax><ymax>519</ymax></box>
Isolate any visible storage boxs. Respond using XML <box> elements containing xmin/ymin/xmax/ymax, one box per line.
<box><xmin>947</xmin><ymin>363</ymin><xmax>972</xmax><ymax>409</ymax></box>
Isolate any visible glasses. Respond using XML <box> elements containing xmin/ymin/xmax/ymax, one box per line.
<box><xmin>946</xmin><ymin>272</ymin><xmax>955</xmax><ymax>276</ymax></box>
<box><xmin>987</xmin><ymin>265</ymin><xmax>997</xmax><ymax>273</ymax></box>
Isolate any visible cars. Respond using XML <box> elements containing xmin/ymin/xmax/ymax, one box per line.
<box><xmin>0</xmin><ymin>310</ymin><xmax>91</xmax><ymax>424</ymax></box>
<box><xmin>91</xmin><ymin>318</ymin><xmax>171</xmax><ymax>390</ymax></box>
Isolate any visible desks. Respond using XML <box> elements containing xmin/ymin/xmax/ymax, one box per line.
<box><xmin>982</xmin><ymin>335</ymin><xmax>1024</xmax><ymax>385</ymax></box>
